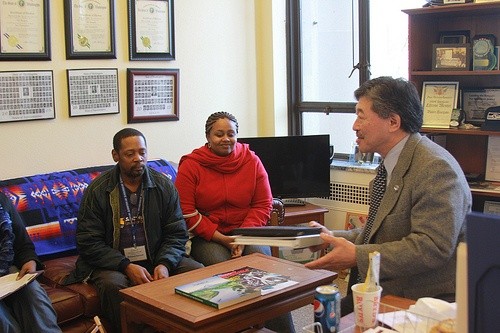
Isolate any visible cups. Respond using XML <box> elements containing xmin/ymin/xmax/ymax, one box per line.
<box><xmin>350</xmin><ymin>282</ymin><xmax>384</xmax><ymax>328</ymax></box>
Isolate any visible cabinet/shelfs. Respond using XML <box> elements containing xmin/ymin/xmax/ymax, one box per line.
<box><xmin>401</xmin><ymin>3</ymin><xmax>500</xmax><ymax>198</ymax></box>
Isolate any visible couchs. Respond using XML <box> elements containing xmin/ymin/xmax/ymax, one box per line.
<box><xmin>0</xmin><ymin>159</ymin><xmax>285</xmax><ymax>333</ymax></box>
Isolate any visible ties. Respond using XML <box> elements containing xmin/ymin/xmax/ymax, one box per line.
<box><xmin>363</xmin><ymin>161</ymin><xmax>386</xmax><ymax>244</ymax></box>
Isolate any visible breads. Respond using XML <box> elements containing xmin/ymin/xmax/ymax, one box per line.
<box><xmin>428</xmin><ymin>318</ymin><xmax>457</xmax><ymax>333</ymax></box>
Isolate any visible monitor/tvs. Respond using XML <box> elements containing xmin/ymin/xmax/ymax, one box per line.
<box><xmin>236</xmin><ymin>134</ymin><xmax>330</xmax><ymax>199</ymax></box>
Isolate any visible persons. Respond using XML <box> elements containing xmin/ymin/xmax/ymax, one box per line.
<box><xmin>64</xmin><ymin>127</ymin><xmax>204</xmax><ymax>333</ymax></box>
<box><xmin>0</xmin><ymin>193</ymin><xmax>62</xmax><ymax>333</ymax></box>
<box><xmin>174</xmin><ymin>112</ymin><xmax>296</xmax><ymax>333</ymax></box>
<box><xmin>305</xmin><ymin>76</ymin><xmax>474</xmax><ymax>318</ymax></box>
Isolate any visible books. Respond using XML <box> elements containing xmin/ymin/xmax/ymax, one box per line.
<box><xmin>175</xmin><ymin>267</ymin><xmax>298</xmax><ymax>309</ymax></box>
<box><xmin>229</xmin><ymin>226</ymin><xmax>323</xmax><ymax>249</ymax></box>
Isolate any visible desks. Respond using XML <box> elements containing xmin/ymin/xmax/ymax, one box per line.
<box><xmin>338</xmin><ymin>294</ymin><xmax>416</xmax><ymax>333</ymax></box>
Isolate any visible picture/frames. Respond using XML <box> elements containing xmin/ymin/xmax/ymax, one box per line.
<box><xmin>439</xmin><ymin>29</ymin><xmax>471</xmax><ymax>44</ymax></box>
<box><xmin>127</xmin><ymin>0</ymin><xmax>177</xmax><ymax>62</ymax></box>
<box><xmin>66</xmin><ymin>68</ymin><xmax>120</xmax><ymax>118</ymax></box>
<box><xmin>0</xmin><ymin>0</ymin><xmax>51</xmax><ymax>61</ymax></box>
<box><xmin>431</xmin><ymin>43</ymin><xmax>471</xmax><ymax>71</ymax></box>
<box><xmin>126</xmin><ymin>68</ymin><xmax>180</xmax><ymax>123</ymax></box>
<box><xmin>420</xmin><ymin>81</ymin><xmax>460</xmax><ymax>128</ymax></box>
<box><xmin>0</xmin><ymin>69</ymin><xmax>56</xmax><ymax>124</ymax></box>
<box><xmin>459</xmin><ymin>87</ymin><xmax>500</xmax><ymax>123</ymax></box>
<box><xmin>63</xmin><ymin>0</ymin><xmax>117</xmax><ymax>60</ymax></box>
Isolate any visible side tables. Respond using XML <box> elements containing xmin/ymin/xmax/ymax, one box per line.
<box><xmin>278</xmin><ymin>203</ymin><xmax>330</xmax><ymax>258</ymax></box>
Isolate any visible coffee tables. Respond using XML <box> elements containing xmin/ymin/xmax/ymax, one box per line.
<box><xmin>118</xmin><ymin>252</ymin><xmax>338</xmax><ymax>333</ymax></box>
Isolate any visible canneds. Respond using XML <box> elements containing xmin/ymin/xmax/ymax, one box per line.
<box><xmin>314</xmin><ymin>286</ymin><xmax>341</xmax><ymax>333</ymax></box>
